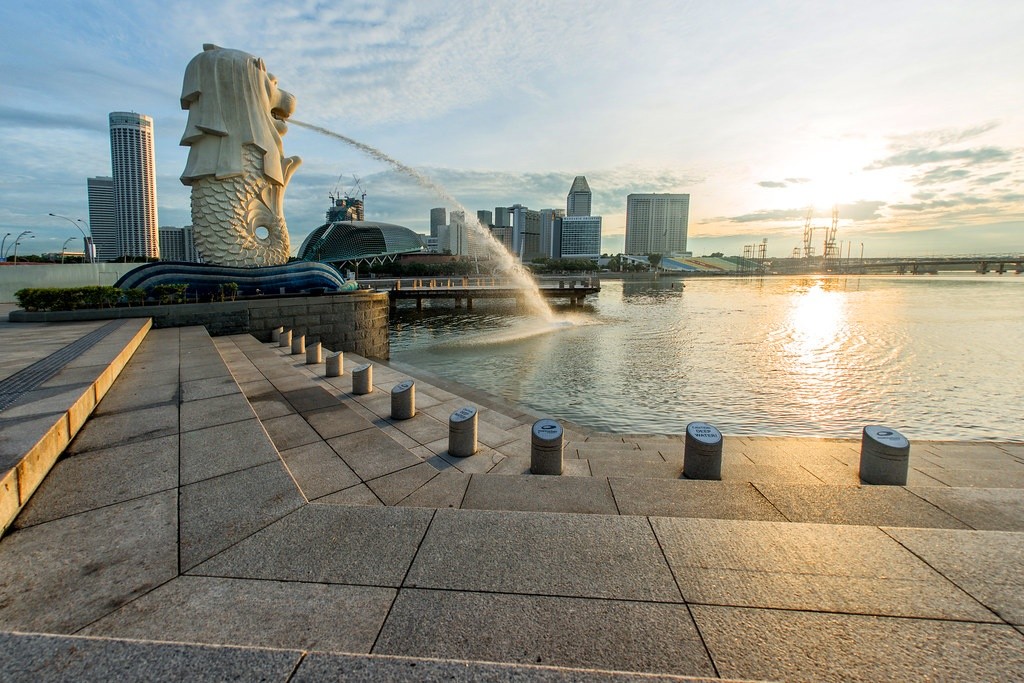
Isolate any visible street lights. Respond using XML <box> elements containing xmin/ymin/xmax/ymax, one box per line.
<box><xmin>50</xmin><ymin>212</ymin><xmax>92</xmax><ymax>264</ymax></box>
<box><xmin>5</xmin><ymin>236</ymin><xmax>35</xmax><ymax>262</ymax></box>
<box><xmin>1</xmin><ymin>232</ymin><xmax>11</xmax><ymax>261</ymax></box>
<box><xmin>78</xmin><ymin>219</ymin><xmax>95</xmax><ymax>264</ymax></box>
